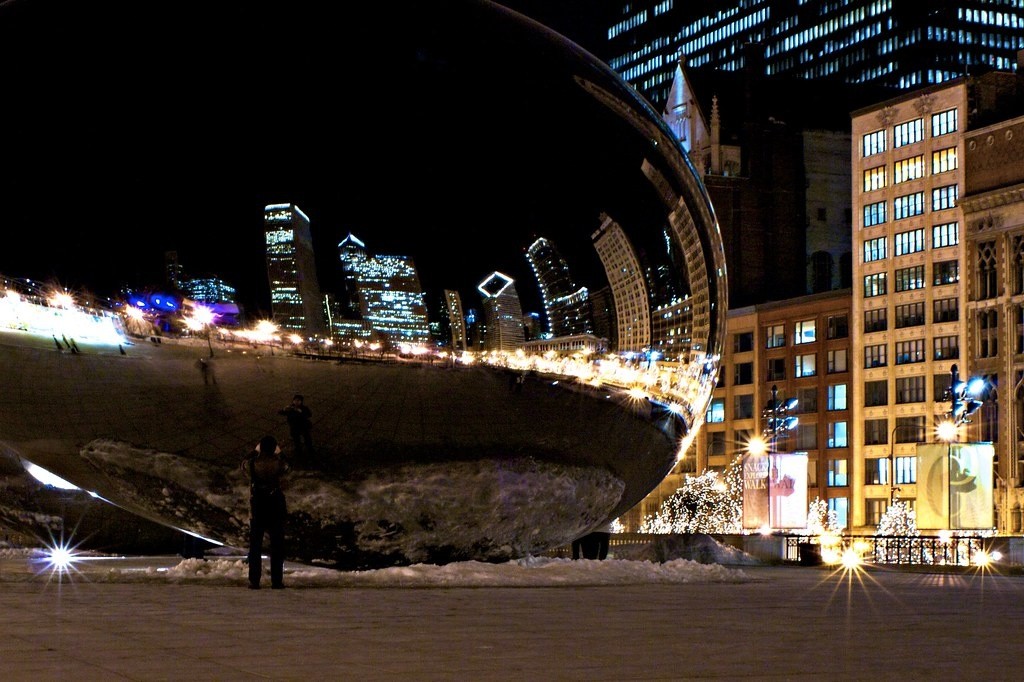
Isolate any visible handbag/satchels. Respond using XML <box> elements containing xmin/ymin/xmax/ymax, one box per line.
<box><xmin>249</xmin><ymin>478</ymin><xmax>276</xmax><ymax>506</ymax></box>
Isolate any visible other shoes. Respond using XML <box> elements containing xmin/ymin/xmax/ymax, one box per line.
<box><xmin>248</xmin><ymin>583</ymin><xmax>259</xmax><ymax>589</ymax></box>
<box><xmin>271</xmin><ymin>584</ymin><xmax>284</xmax><ymax>588</ymax></box>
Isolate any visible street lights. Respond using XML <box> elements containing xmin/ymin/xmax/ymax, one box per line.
<box><xmin>891</xmin><ymin>422</ymin><xmax>957</xmax><ymax>507</ymax></box>
<box><xmin>185</xmin><ymin>306</ymin><xmax>214</xmax><ymax>359</ymax></box>
<box><xmin>942</xmin><ymin>363</ymin><xmax>988</xmax><ymax>531</ymax></box>
<box><xmin>259</xmin><ymin>320</ymin><xmax>280</xmax><ymax>357</ymax></box>
<box><xmin>705</xmin><ymin>440</ymin><xmax>766</xmax><ymax>472</ymax></box>
<box><xmin>761</xmin><ymin>385</ymin><xmax>799</xmax><ymax>534</ymax></box>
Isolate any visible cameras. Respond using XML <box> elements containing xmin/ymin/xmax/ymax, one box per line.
<box><xmin>290</xmin><ymin>403</ymin><xmax>296</xmax><ymax>410</ymax></box>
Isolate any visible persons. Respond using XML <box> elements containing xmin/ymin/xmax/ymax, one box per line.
<box><xmin>194</xmin><ymin>358</ymin><xmax>217</xmax><ymax>386</ymax></box>
<box><xmin>278</xmin><ymin>394</ymin><xmax>312</xmax><ymax>447</ymax></box>
<box><xmin>239</xmin><ymin>438</ymin><xmax>293</xmax><ymax>589</ymax></box>
<box><xmin>572</xmin><ymin>525</ymin><xmax>611</xmax><ymax>561</ymax></box>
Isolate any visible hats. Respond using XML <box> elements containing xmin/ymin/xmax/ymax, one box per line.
<box><xmin>260</xmin><ymin>436</ymin><xmax>277</xmax><ymax>452</ymax></box>
